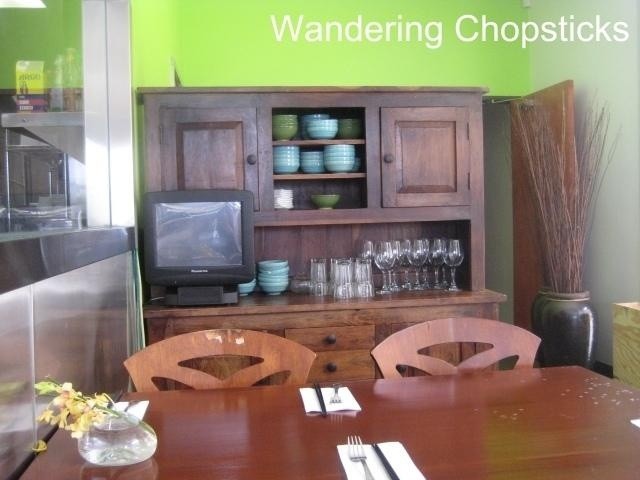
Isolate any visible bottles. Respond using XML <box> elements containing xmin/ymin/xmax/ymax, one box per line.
<box><xmin>46</xmin><ymin>45</ymin><xmax>77</xmax><ymax>113</ymax></box>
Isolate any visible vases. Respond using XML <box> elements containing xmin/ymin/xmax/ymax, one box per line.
<box><xmin>76</xmin><ymin>412</ymin><xmax>158</xmax><ymax>468</ymax></box>
<box><xmin>531</xmin><ymin>289</ymin><xmax>600</xmax><ymax>373</ymax></box>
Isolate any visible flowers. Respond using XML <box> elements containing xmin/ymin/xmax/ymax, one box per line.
<box><xmin>31</xmin><ymin>378</ymin><xmax>139</xmax><ymax>460</ymax></box>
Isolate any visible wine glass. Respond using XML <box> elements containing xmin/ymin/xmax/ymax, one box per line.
<box><xmin>355</xmin><ymin>236</ymin><xmax>465</xmax><ymax>295</ymax></box>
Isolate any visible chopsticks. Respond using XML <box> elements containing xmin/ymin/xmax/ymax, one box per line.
<box><xmin>312</xmin><ymin>382</ymin><xmax>328</xmax><ymax>417</ymax></box>
<box><xmin>369</xmin><ymin>440</ymin><xmax>400</xmax><ymax>480</ymax></box>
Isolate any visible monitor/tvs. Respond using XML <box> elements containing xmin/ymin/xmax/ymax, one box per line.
<box><xmin>141</xmin><ymin>189</ymin><xmax>255</xmax><ymax>307</ymax></box>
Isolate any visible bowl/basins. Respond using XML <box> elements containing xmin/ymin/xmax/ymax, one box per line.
<box><xmin>255</xmin><ymin>258</ymin><xmax>290</xmax><ymax>296</ymax></box>
<box><xmin>273</xmin><ymin>186</ymin><xmax>294</xmax><ymax>210</ymax></box>
<box><xmin>271</xmin><ymin>144</ymin><xmax>362</xmax><ymax>174</ymax></box>
<box><xmin>235</xmin><ymin>278</ymin><xmax>258</xmax><ymax>297</ymax></box>
<box><xmin>272</xmin><ymin>113</ymin><xmax>363</xmax><ymax>141</ymax></box>
<box><xmin>307</xmin><ymin>192</ymin><xmax>341</xmax><ymax>210</ymax></box>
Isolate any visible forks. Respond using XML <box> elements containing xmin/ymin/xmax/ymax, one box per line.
<box><xmin>345</xmin><ymin>434</ymin><xmax>375</xmax><ymax>480</ymax></box>
<box><xmin>329</xmin><ymin>382</ymin><xmax>342</xmax><ymax>404</ymax></box>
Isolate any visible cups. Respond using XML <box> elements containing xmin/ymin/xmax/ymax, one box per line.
<box><xmin>309</xmin><ymin>254</ymin><xmax>375</xmax><ymax>299</ymax></box>
